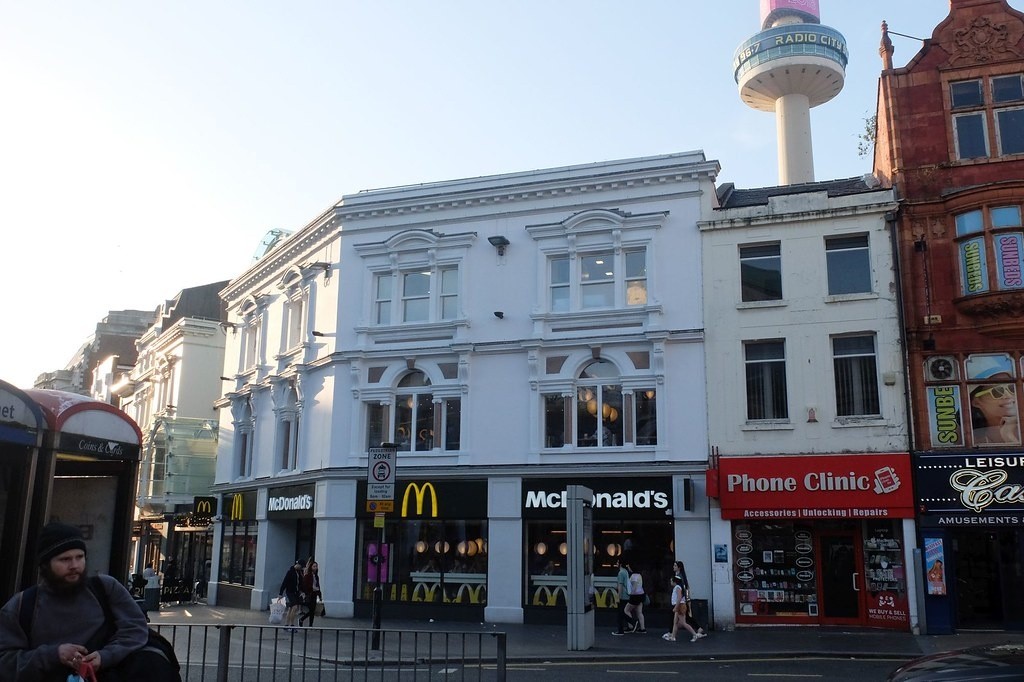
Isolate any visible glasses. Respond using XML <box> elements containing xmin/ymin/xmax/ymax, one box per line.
<box><xmin>973</xmin><ymin>383</ymin><xmax>1015</xmax><ymax>399</ymax></box>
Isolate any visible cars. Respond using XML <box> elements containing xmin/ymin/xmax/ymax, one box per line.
<box><xmin>888</xmin><ymin>643</ymin><xmax>1024</xmax><ymax>682</ymax></box>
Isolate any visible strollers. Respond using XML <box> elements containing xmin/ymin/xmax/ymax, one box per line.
<box><xmin>128</xmin><ymin>580</ymin><xmax>150</xmax><ymax>623</ymax></box>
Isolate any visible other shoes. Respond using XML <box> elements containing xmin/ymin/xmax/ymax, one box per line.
<box><xmin>697</xmin><ymin>630</ymin><xmax>707</xmax><ymax>638</ymax></box>
<box><xmin>662</xmin><ymin>633</ymin><xmax>672</xmax><ymax>639</ymax></box>
<box><xmin>290</xmin><ymin>625</ymin><xmax>297</xmax><ymax>633</ymax></box>
<box><xmin>283</xmin><ymin>625</ymin><xmax>291</xmax><ymax>633</ymax></box>
<box><xmin>309</xmin><ymin>624</ymin><xmax>313</xmax><ymax>630</ymax></box>
<box><xmin>624</xmin><ymin>627</ymin><xmax>633</xmax><ymax>633</ymax></box>
<box><xmin>299</xmin><ymin>619</ymin><xmax>303</xmax><ymax>627</ymax></box>
<box><xmin>665</xmin><ymin>637</ymin><xmax>676</xmax><ymax>642</ymax></box>
<box><xmin>690</xmin><ymin>635</ymin><xmax>699</xmax><ymax>642</ymax></box>
<box><xmin>632</xmin><ymin>620</ymin><xmax>638</xmax><ymax>633</ymax></box>
<box><xmin>636</xmin><ymin>629</ymin><xmax>647</xmax><ymax>634</ymax></box>
<box><xmin>612</xmin><ymin>631</ymin><xmax>624</xmax><ymax>636</ymax></box>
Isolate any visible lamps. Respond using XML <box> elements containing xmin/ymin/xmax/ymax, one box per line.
<box><xmin>607</xmin><ymin>544</ymin><xmax>621</xmax><ymax>557</ymax></box>
<box><xmin>487</xmin><ymin>235</ymin><xmax>511</xmax><ymax>256</ymax></box>
<box><xmin>579</xmin><ymin>388</ymin><xmax>593</xmax><ymax>402</ymax></box>
<box><xmin>494</xmin><ymin>311</ymin><xmax>503</xmax><ymax>319</ymax></box>
<box><xmin>586</xmin><ymin>398</ymin><xmax>597</xmax><ymax>415</ymax></box>
<box><xmin>604</xmin><ymin>408</ymin><xmax>618</xmax><ymax>422</ymax></box>
<box><xmin>643</xmin><ymin>391</ymin><xmax>655</xmax><ymax>399</ymax></box>
<box><xmin>407</xmin><ymin>396</ymin><xmax>419</xmax><ymax>409</ymax></box>
<box><xmin>595</xmin><ymin>404</ymin><xmax>611</xmax><ymax>419</ymax></box>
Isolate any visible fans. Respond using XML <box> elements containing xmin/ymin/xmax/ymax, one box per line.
<box><xmin>923</xmin><ymin>356</ymin><xmax>961</xmax><ymax>383</ymax></box>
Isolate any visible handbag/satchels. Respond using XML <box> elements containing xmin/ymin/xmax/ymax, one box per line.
<box><xmin>269</xmin><ymin>600</ymin><xmax>286</xmax><ymax>624</ymax></box>
<box><xmin>642</xmin><ymin>594</ymin><xmax>650</xmax><ymax>606</ymax></box>
<box><xmin>314</xmin><ymin>600</ymin><xmax>326</xmax><ymax>617</ymax></box>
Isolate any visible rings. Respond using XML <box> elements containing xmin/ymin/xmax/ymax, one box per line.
<box><xmin>71</xmin><ymin>658</ymin><xmax>76</xmax><ymax>662</ymax></box>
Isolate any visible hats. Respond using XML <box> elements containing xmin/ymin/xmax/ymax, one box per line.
<box><xmin>298</xmin><ymin>559</ymin><xmax>306</xmax><ymax>568</ymax></box>
<box><xmin>966</xmin><ymin>357</ymin><xmax>1013</xmax><ymax>394</ymax></box>
<box><xmin>37</xmin><ymin>523</ymin><xmax>87</xmax><ymax>567</ymax></box>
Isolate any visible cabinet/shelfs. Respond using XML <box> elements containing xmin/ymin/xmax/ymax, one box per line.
<box><xmin>866</xmin><ymin>549</ymin><xmax>902</xmax><ymax>590</ymax></box>
<box><xmin>737</xmin><ymin>536</ymin><xmax>816</xmax><ymax>605</ymax></box>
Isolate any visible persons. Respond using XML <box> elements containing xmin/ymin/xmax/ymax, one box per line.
<box><xmin>593</xmin><ymin>421</ymin><xmax>612</xmax><ymax>447</ymax></box>
<box><xmin>927</xmin><ymin>560</ymin><xmax>944</xmax><ymax>594</ymax></box>
<box><xmin>835</xmin><ymin>543</ymin><xmax>859</xmax><ymax>616</ymax></box>
<box><xmin>0</xmin><ymin>522</ymin><xmax>182</xmax><ymax>682</ymax></box>
<box><xmin>661</xmin><ymin>561</ymin><xmax>707</xmax><ymax>640</ymax></box>
<box><xmin>968</xmin><ymin>353</ymin><xmax>1017</xmax><ymax>445</ymax></box>
<box><xmin>419</xmin><ymin>556</ymin><xmax>487</xmax><ymax>598</ymax></box>
<box><xmin>610</xmin><ymin>556</ymin><xmax>635</xmax><ymax>635</ymax></box>
<box><xmin>665</xmin><ymin>576</ymin><xmax>697</xmax><ymax>643</ymax></box>
<box><xmin>623</xmin><ymin>560</ymin><xmax>647</xmax><ymax>633</ymax></box>
<box><xmin>296</xmin><ymin>560</ymin><xmax>322</xmax><ymax>630</ymax></box>
<box><xmin>280</xmin><ymin>559</ymin><xmax>305</xmax><ymax>632</ymax></box>
<box><xmin>128</xmin><ymin>554</ymin><xmax>183</xmax><ymax>608</ymax></box>
<box><xmin>533</xmin><ymin>543</ymin><xmax>557</xmax><ymax>602</ymax></box>
